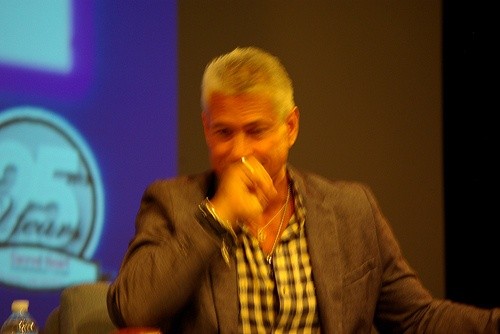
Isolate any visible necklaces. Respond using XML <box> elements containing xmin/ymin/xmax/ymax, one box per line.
<box><xmin>267</xmin><ymin>185</ymin><xmax>290</xmax><ymax>262</ymax></box>
<box><xmin>246</xmin><ymin>186</ymin><xmax>288</xmax><ymax>241</ymax></box>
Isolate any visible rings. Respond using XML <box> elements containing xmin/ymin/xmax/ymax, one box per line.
<box><xmin>240</xmin><ymin>155</ymin><xmax>252</xmax><ymax>174</ymax></box>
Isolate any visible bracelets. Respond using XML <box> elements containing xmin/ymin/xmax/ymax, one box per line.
<box><xmin>195</xmin><ymin>193</ymin><xmax>239</xmax><ymax>267</ymax></box>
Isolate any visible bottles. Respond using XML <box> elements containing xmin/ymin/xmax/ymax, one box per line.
<box><xmin>0</xmin><ymin>299</ymin><xmax>39</xmax><ymax>334</ymax></box>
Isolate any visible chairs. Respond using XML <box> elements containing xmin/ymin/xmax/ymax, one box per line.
<box><xmin>43</xmin><ymin>280</ymin><xmax>128</xmax><ymax>333</ymax></box>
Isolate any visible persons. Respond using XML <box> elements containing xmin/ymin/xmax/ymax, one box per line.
<box><xmin>78</xmin><ymin>0</ymin><xmax>500</xmax><ymax>334</ymax></box>
